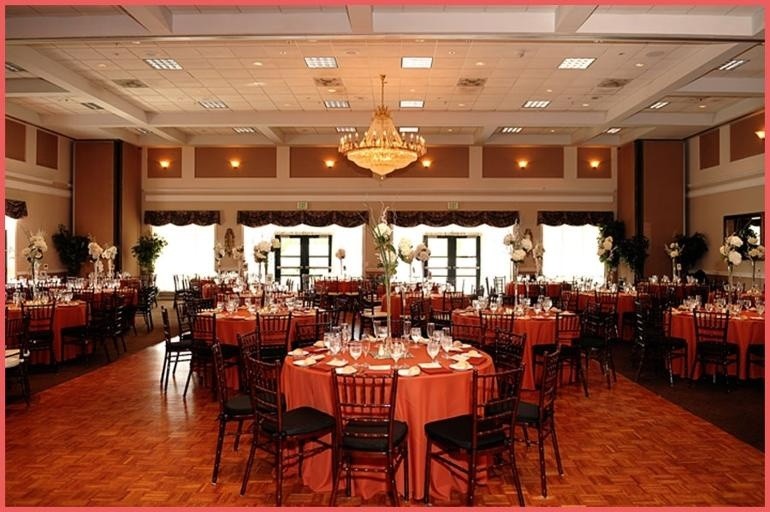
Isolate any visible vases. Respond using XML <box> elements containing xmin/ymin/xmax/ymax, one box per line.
<box><xmin>385</xmin><ymin>262</ymin><xmax>425</xmax><ymax>341</ymax></box>
<box><xmin>93</xmin><ymin>260</ymin><xmax>113</xmax><ymax>288</ymax></box>
<box><xmin>258</xmin><ymin>260</ymin><xmax>268</xmax><ymax>284</ymax></box>
<box><xmin>727</xmin><ymin>260</ymin><xmax>756</xmax><ymax>290</ymax></box>
<box><xmin>217</xmin><ymin>263</ymin><xmax>242</xmax><ymax>282</ymax></box>
<box><xmin>30</xmin><ymin>261</ymin><xmax>39</xmax><ymax>299</ymax></box>
<box><xmin>514</xmin><ymin>263</ymin><xmax>518</xmax><ymax>313</ymax></box>
<box><xmin>672</xmin><ymin>258</ymin><xmax>677</xmax><ymax>283</ymax></box>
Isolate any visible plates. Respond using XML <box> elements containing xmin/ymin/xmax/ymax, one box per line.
<box><xmin>453</xmin><ymin>307</ymin><xmax>570</xmax><ymax>320</ymax></box>
<box><xmin>287</xmin><ymin>337</ymin><xmax>483</xmax><ymax>377</ymax></box>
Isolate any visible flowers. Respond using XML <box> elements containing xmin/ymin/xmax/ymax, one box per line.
<box><xmin>664</xmin><ymin>240</ymin><xmax>683</xmax><ymax>257</ymax></box>
<box><xmin>502</xmin><ymin>218</ymin><xmax>545</xmax><ymax>279</ymax></box>
<box><xmin>20</xmin><ymin>225</ymin><xmax>50</xmax><ymax>264</ymax></box>
<box><xmin>355</xmin><ymin>198</ymin><xmax>431</xmax><ymax>297</ymax></box>
<box><xmin>597</xmin><ymin>236</ymin><xmax>619</xmax><ymax>267</ymax></box>
<box><xmin>719</xmin><ymin>222</ymin><xmax>765</xmax><ymax>279</ymax></box>
<box><xmin>212</xmin><ymin>237</ymin><xmax>247</xmax><ymax>267</ymax></box>
<box><xmin>88</xmin><ymin>235</ymin><xmax>118</xmax><ymax>260</ymax></box>
<box><xmin>254</xmin><ymin>238</ymin><xmax>281</xmax><ymax>262</ymax></box>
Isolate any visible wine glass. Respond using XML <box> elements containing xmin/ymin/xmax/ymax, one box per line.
<box><xmin>324</xmin><ymin>320</ymin><xmax>453</xmax><ymax>370</ymax></box>
<box><xmin>214</xmin><ymin>267</ymin><xmax>303</xmax><ymax>317</ymax></box>
<box><xmin>6</xmin><ymin>270</ymin><xmax>132</xmax><ymax>305</ymax></box>
<box><xmin>683</xmin><ymin>279</ymin><xmax>764</xmax><ymax>319</ymax></box>
<box><xmin>472</xmin><ymin>293</ymin><xmax>552</xmax><ymax>317</ymax></box>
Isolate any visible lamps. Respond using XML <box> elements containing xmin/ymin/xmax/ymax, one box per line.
<box><xmin>338</xmin><ymin>74</ymin><xmax>427</xmax><ymax>179</ymax></box>
